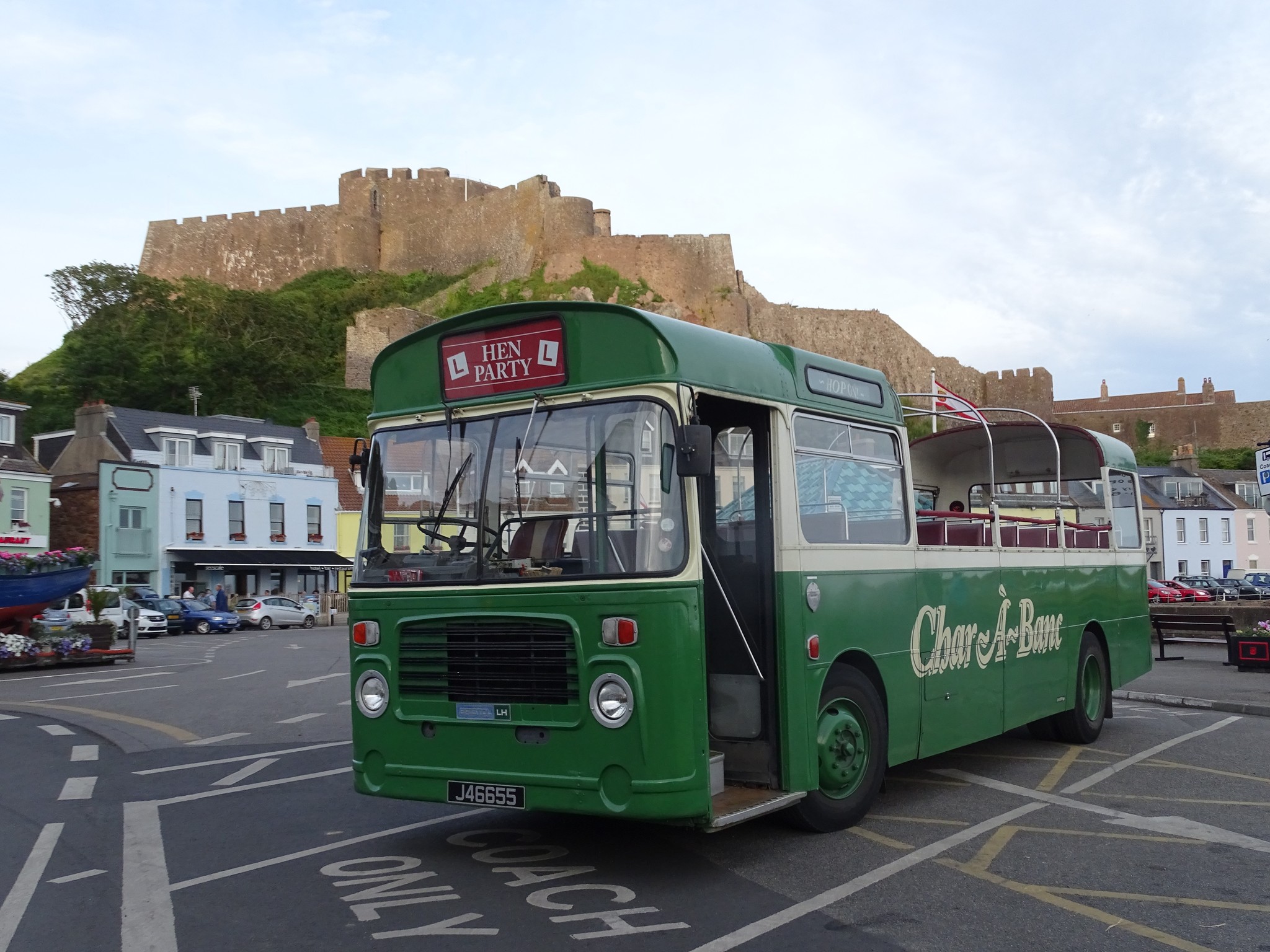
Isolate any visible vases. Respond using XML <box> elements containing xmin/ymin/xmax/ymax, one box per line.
<box><xmin>1231</xmin><ymin>635</ymin><xmax>1270</xmax><ymax>674</ymax></box>
<box><xmin>230</xmin><ymin>535</ymin><xmax>245</xmax><ymax>541</ymax></box>
<box><xmin>0</xmin><ymin>561</ymin><xmax>94</xmax><ymax>575</ymax></box>
<box><xmin>187</xmin><ymin>533</ymin><xmax>203</xmax><ymax>539</ymax></box>
<box><xmin>308</xmin><ymin>538</ymin><xmax>321</xmax><ymax>542</ymax></box>
<box><xmin>271</xmin><ymin>536</ymin><xmax>286</xmax><ymax>542</ymax></box>
<box><xmin>75</xmin><ymin>624</ymin><xmax>114</xmax><ymax>650</ymax></box>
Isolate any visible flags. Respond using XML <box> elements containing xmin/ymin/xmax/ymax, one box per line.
<box><xmin>935</xmin><ymin>380</ymin><xmax>991</xmax><ymax>423</ymax></box>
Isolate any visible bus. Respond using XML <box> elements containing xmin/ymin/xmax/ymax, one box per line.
<box><xmin>347</xmin><ymin>298</ymin><xmax>1155</xmax><ymax>836</ymax></box>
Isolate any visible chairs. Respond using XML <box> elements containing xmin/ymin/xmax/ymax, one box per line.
<box><xmin>506</xmin><ymin>519</ymin><xmax>570</xmax><ymax>559</ymax></box>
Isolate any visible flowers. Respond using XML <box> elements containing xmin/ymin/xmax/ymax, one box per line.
<box><xmin>0</xmin><ymin>632</ymin><xmax>92</xmax><ymax>659</ymax></box>
<box><xmin>309</xmin><ymin>534</ymin><xmax>324</xmax><ymax>539</ymax></box>
<box><xmin>69</xmin><ymin>585</ymin><xmax>136</xmax><ymax>645</ymax></box>
<box><xmin>232</xmin><ymin>532</ymin><xmax>247</xmax><ymax>538</ymax></box>
<box><xmin>270</xmin><ymin>534</ymin><xmax>286</xmax><ymax>539</ymax></box>
<box><xmin>1236</xmin><ymin>620</ymin><xmax>1270</xmax><ymax>637</ymax></box>
<box><xmin>189</xmin><ymin>532</ymin><xmax>204</xmax><ymax>536</ymax></box>
<box><xmin>0</xmin><ymin>546</ymin><xmax>101</xmax><ymax>571</ymax></box>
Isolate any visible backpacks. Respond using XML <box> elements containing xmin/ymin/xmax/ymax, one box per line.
<box><xmin>202</xmin><ymin>594</ymin><xmax>213</xmax><ymax>607</ymax></box>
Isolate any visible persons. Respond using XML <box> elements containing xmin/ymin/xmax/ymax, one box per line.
<box><xmin>264</xmin><ymin>590</ymin><xmax>343</xmax><ymax>614</ymax></box>
<box><xmin>184</xmin><ymin>584</ymin><xmax>228</xmax><ymax>612</ymax></box>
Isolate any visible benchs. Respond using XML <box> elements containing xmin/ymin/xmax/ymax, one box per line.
<box><xmin>1150</xmin><ymin>614</ymin><xmax>1238</xmax><ymax>665</ymax></box>
<box><xmin>571</xmin><ymin>502</ymin><xmax>1123</xmax><ymax>575</ymax></box>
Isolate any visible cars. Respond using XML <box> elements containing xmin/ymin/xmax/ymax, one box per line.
<box><xmin>1157</xmin><ymin>580</ymin><xmax>1212</xmax><ymax>602</ymax></box>
<box><xmin>163</xmin><ymin>598</ymin><xmax>242</xmax><ymax>635</ymax></box>
<box><xmin>1147</xmin><ymin>578</ymin><xmax>1182</xmax><ymax>603</ymax></box>
<box><xmin>233</xmin><ymin>595</ymin><xmax>318</xmax><ymax>631</ymax></box>
<box><xmin>1173</xmin><ymin>575</ymin><xmax>1270</xmax><ymax>602</ymax></box>
<box><xmin>31</xmin><ymin>585</ymin><xmax>187</xmax><ymax>640</ymax></box>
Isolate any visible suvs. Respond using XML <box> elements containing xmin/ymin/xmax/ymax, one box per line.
<box><xmin>1244</xmin><ymin>572</ymin><xmax>1270</xmax><ymax>589</ymax></box>
